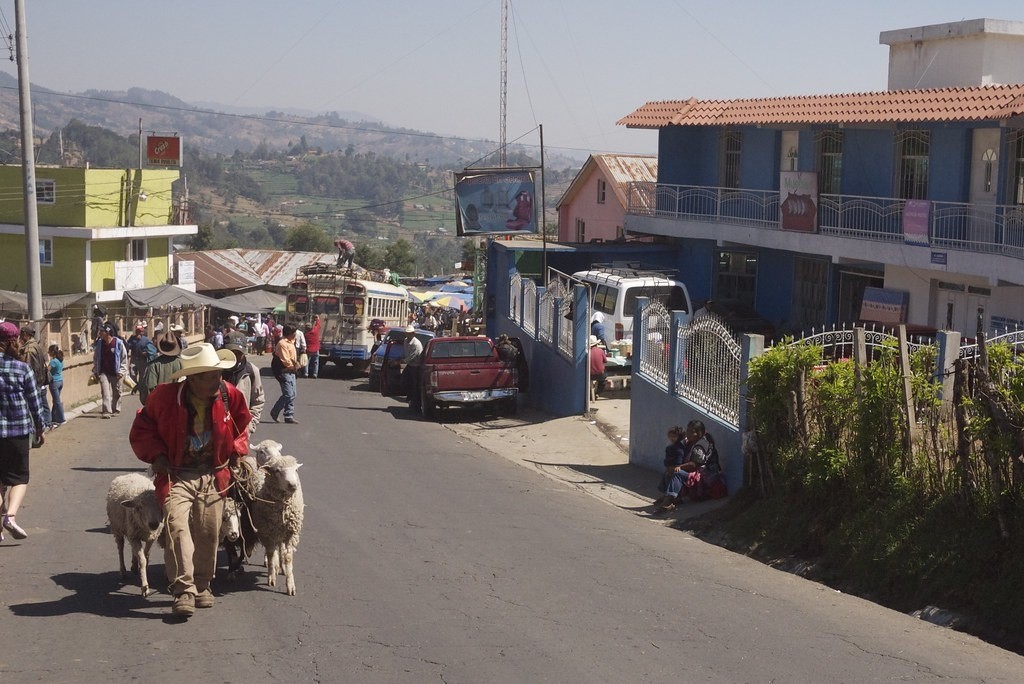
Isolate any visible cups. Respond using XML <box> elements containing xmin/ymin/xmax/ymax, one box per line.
<box><xmin>627</xmin><ymin>353</ymin><xmax>630</xmax><ymax>359</ymax></box>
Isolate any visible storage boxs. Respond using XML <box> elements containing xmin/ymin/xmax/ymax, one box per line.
<box><xmin>604</xmin><ymin>341</ymin><xmax>632</xmax><ymax>390</ymax></box>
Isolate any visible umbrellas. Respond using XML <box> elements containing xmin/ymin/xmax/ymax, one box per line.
<box><xmin>408</xmin><ymin>291</ymin><xmax>473</xmax><ymax>313</ymax></box>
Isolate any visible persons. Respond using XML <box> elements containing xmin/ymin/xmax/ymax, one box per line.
<box><xmin>591</xmin><ymin>311</ymin><xmax>605</xmax><ymax>342</ymax></box>
<box><xmin>495</xmin><ymin>334</ymin><xmax>519</xmax><ymax>415</ymax></box>
<box><xmin>652</xmin><ymin>419</ymin><xmax>727</xmax><ymax>511</ymax></box>
<box><xmin>213</xmin><ymin>315</ymin><xmax>283</xmax><ymax>356</ymax></box>
<box><xmin>591</xmin><ymin>335</ymin><xmax>608</xmax><ymax>398</ymax></box>
<box><xmin>296</xmin><ymin>330</ymin><xmax>306</xmax><ymax>379</ymax></box>
<box><xmin>334</xmin><ymin>240</ymin><xmax>355</xmax><ymax>268</ymax></box>
<box><xmin>649</xmin><ymin>332</ymin><xmax>687</xmax><ymax>375</ymax></box>
<box><xmin>139</xmin><ymin>331</ymin><xmax>182</xmax><ymax>406</ymax></box>
<box><xmin>0</xmin><ymin>321</ymin><xmax>64</xmax><ymax>539</ymax></box>
<box><xmin>304</xmin><ymin>315</ymin><xmax>320</xmax><ymax>379</ymax></box>
<box><xmin>270</xmin><ymin>323</ymin><xmax>301</xmax><ymax>424</ymax></box>
<box><xmin>408</xmin><ymin>302</ymin><xmax>443</xmax><ymax>332</ymax></box>
<box><xmin>92</xmin><ymin>324</ymin><xmax>127</xmax><ymax>419</ymax></box>
<box><xmin>222</xmin><ymin>331</ymin><xmax>265</xmax><ymax>438</ymax></box>
<box><xmin>129</xmin><ymin>343</ymin><xmax>252</xmax><ymax>616</ymax></box>
<box><xmin>391</xmin><ymin>325</ymin><xmax>423</xmax><ymax>413</ymax></box>
<box><xmin>105</xmin><ymin>320</ymin><xmax>188</xmax><ymax>394</ymax></box>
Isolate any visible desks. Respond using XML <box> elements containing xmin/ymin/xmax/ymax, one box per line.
<box><xmin>605</xmin><ymin>359</ymin><xmax>633</xmax><ymax>369</ymax></box>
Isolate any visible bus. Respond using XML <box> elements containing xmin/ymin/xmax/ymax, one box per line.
<box><xmin>284</xmin><ymin>263</ymin><xmax>408</xmax><ymax>368</ymax></box>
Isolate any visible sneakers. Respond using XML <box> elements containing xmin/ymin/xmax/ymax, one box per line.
<box><xmin>173</xmin><ymin>592</ymin><xmax>195</xmax><ymax>615</ymax></box>
<box><xmin>196</xmin><ymin>586</ymin><xmax>214</xmax><ymax>608</ymax></box>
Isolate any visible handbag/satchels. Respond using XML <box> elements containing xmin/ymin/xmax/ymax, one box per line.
<box><xmin>44</xmin><ymin>367</ymin><xmax>52</xmax><ymax>386</ymax></box>
<box><xmin>300</xmin><ymin>353</ymin><xmax>308</xmax><ymax>367</ymax></box>
<box><xmin>687</xmin><ymin>470</ymin><xmax>728</xmax><ymax>501</ymax></box>
<box><xmin>88</xmin><ymin>374</ymin><xmax>98</xmax><ymax>386</ymax></box>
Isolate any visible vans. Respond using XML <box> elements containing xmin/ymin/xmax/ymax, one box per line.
<box><xmin>571</xmin><ymin>263</ymin><xmax>693</xmax><ymax>357</ymax></box>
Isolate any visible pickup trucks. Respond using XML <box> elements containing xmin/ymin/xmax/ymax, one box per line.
<box><xmin>379</xmin><ymin>336</ymin><xmax>529</xmax><ymax>417</ymax></box>
<box><xmin>369</xmin><ymin>328</ymin><xmax>435</xmax><ymax>388</ymax></box>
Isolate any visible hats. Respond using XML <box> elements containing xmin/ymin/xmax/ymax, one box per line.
<box><xmin>283</xmin><ymin>325</ymin><xmax>299</xmax><ymax>336</ymax></box>
<box><xmin>400</xmin><ymin>325</ymin><xmax>418</xmax><ymax>335</ymax></box>
<box><xmin>224</xmin><ymin>332</ymin><xmax>247</xmax><ymax>354</ymax></box>
<box><xmin>591</xmin><ymin>335</ymin><xmax>601</xmax><ymax>346</ymax></box>
<box><xmin>157</xmin><ymin>331</ymin><xmax>180</xmax><ymax>356</ymax></box>
<box><xmin>136</xmin><ymin>328</ymin><xmax>144</xmax><ymax>333</ymax></box>
<box><xmin>171</xmin><ymin>325</ymin><xmax>185</xmax><ymax>332</ymax></box>
<box><xmin>169</xmin><ymin>343</ymin><xmax>237</xmax><ymax>379</ymax></box>
<box><xmin>22</xmin><ymin>326</ymin><xmax>35</xmax><ymax>336</ymax></box>
<box><xmin>0</xmin><ymin>322</ymin><xmax>20</xmax><ymax>340</ymax></box>
<box><xmin>97</xmin><ymin>324</ymin><xmax>114</xmax><ymax>336</ymax></box>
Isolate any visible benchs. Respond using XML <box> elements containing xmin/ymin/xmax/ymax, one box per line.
<box><xmin>591</xmin><ymin>374</ymin><xmax>632</xmax><ymax>401</ymax></box>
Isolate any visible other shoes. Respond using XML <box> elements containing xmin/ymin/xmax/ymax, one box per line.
<box><xmin>285</xmin><ymin>418</ymin><xmax>299</xmax><ymax>424</ymax></box>
<box><xmin>3</xmin><ymin>514</ymin><xmax>28</xmax><ymax>539</ymax></box>
<box><xmin>653</xmin><ymin>498</ymin><xmax>674</xmax><ymax>515</ymax></box>
<box><xmin>112</xmin><ymin>410</ymin><xmax>120</xmax><ymax>416</ymax></box>
<box><xmin>102</xmin><ymin>413</ymin><xmax>111</xmax><ymax>419</ymax></box>
<box><xmin>270</xmin><ymin>411</ymin><xmax>280</xmax><ymax>423</ymax></box>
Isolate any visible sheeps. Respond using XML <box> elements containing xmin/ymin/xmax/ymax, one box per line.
<box><xmin>106</xmin><ymin>474</ymin><xmax>165</xmax><ymax>598</ymax></box>
<box><xmin>157</xmin><ymin>439</ymin><xmax>304</xmax><ymax>596</ymax></box>
